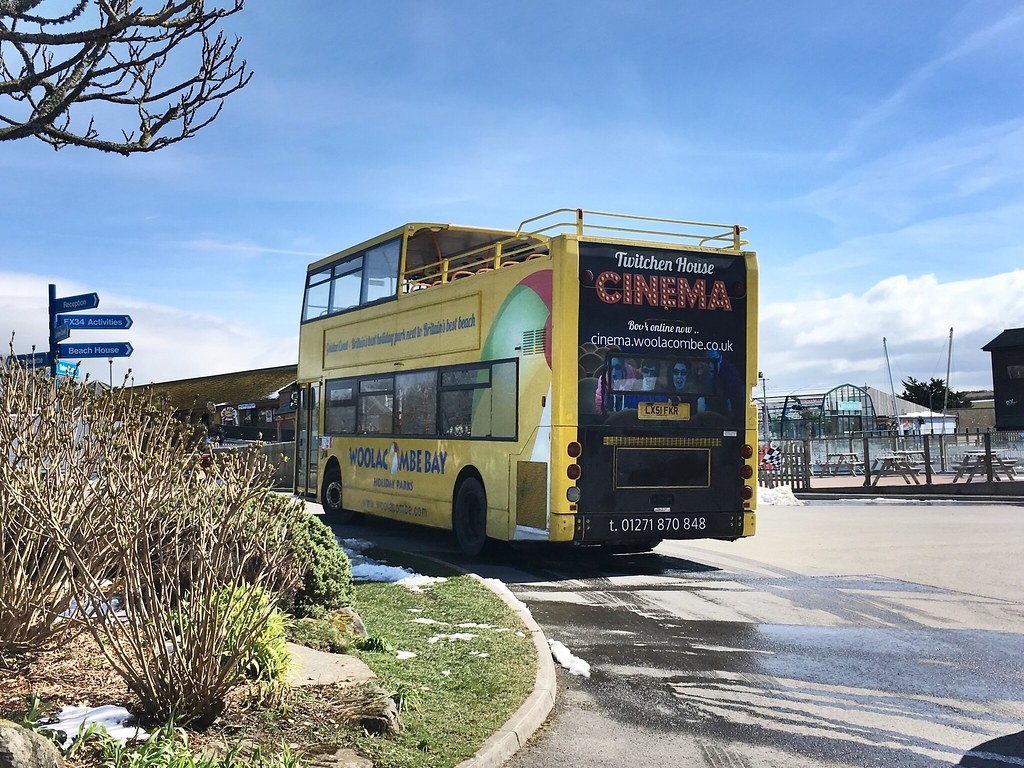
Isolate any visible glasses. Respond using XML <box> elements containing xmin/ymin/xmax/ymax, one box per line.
<box><xmin>641</xmin><ymin>368</ymin><xmax>656</xmax><ymax>376</ymax></box>
<box><xmin>671</xmin><ymin>368</ymin><xmax>688</xmax><ymax>376</ymax></box>
<box><xmin>606</xmin><ymin>363</ymin><xmax>621</xmax><ymax>371</ymax></box>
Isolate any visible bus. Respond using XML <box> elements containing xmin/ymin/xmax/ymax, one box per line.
<box><xmin>292</xmin><ymin>208</ymin><xmax>761</xmax><ymax>571</ymax></box>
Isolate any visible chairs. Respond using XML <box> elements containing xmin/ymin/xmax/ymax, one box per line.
<box><xmin>409</xmin><ymin>254</ymin><xmax>547</xmax><ymax>292</ymax></box>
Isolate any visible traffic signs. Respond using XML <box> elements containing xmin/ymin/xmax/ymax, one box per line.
<box><xmin>6</xmin><ymin>282</ymin><xmax>133</xmax><ymax>373</ymax></box>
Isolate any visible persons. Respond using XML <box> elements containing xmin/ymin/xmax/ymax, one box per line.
<box><xmin>256</xmin><ymin>429</ymin><xmax>263</xmax><ymax>440</ymax></box>
<box><xmin>595</xmin><ymin>348</ymin><xmax>744</xmax><ymax>417</ymax></box>
<box><xmin>219</xmin><ymin>434</ymin><xmax>224</xmax><ymax>443</ymax></box>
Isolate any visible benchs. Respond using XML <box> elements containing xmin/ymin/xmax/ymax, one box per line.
<box><xmin>781</xmin><ymin>449</ymin><xmax>1018</xmax><ymax>487</ymax></box>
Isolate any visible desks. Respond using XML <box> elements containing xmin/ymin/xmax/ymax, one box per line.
<box><xmin>953</xmin><ymin>453</ymin><xmax>1015</xmax><ymax>481</ymax></box>
<box><xmin>819</xmin><ymin>453</ymin><xmax>866</xmax><ymax>477</ymax></box>
<box><xmin>859</xmin><ymin>456</ymin><xmax>920</xmax><ymax>486</ymax></box>
<box><xmin>964</xmin><ymin>449</ymin><xmax>1009</xmax><ymax>453</ymax></box>
<box><xmin>893</xmin><ymin>450</ymin><xmax>936</xmax><ymax>476</ymax></box>
